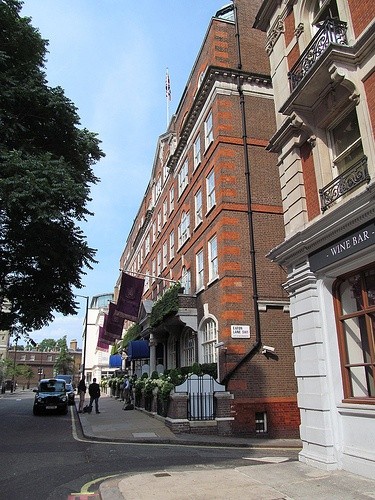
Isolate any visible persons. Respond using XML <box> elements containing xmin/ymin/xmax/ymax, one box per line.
<box><xmin>88</xmin><ymin>378</ymin><xmax>102</xmax><ymax>413</ymax></box>
<box><xmin>122</xmin><ymin>375</ymin><xmax>134</xmax><ymax>406</ymax></box>
<box><xmin>77</xmin><ymin>375</ymin><xmax>87</xmax><ymax>413</ymax></box>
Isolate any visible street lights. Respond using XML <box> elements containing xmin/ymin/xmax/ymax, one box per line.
<box><xmin>74</xmin><ymin>294</ymin><xmax>89</xmax><ymax>376</ymax></box>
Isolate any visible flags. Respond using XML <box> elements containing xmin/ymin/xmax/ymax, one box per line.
<box><xmin>98</xmin><ymin>314</ymin><xmax>116</xmax><ymax>347</ymax></box>
<box><xmin>95</xmin><ymin>326</ymin><xmax>110</xmax><ymax>353</ymax></box>
<box><xmin>113</xmin><ymin>273</ymin><xmax>145</xmax><ymax>324</ymax></box>
<box><xmin>104</xmin><ymin>301</ymin><xmax>125</xmax><ymax>341</ymax></box>
<box><xmin>165</xmin><ymin>71</ymin><xmax>172</xmax><ymax>102</ymax></box>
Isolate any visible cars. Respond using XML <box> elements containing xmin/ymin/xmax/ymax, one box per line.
<box><xmin>66</xmin><ymin>384</ymin><xmax>76</xmax><ymax>405</ymax></box>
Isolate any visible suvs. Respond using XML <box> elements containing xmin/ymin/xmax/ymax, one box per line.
<box><xmin>56</xmin><ymin>375</ymin><xmax>71</xmax><ymax>384</ymax></box>
<box><xmin>33</xmin><ymin>378</ymin><xmax>70</xmax><ymax>415</ymax></box>
<box><xmin>5</xmin><ymin>379</ymin><xmax>18</xmax><ymax>391</ymax></box>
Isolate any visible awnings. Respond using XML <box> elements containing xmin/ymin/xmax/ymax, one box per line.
<box><xmin>109</xmin><ymin>354</ymin><xmax>123</xmax><ymax>370</ymax></box>
<box><xmin>128</xmin><ymin>340</ymin><xmax>151</xmax><ymax>360</ymax></box>
